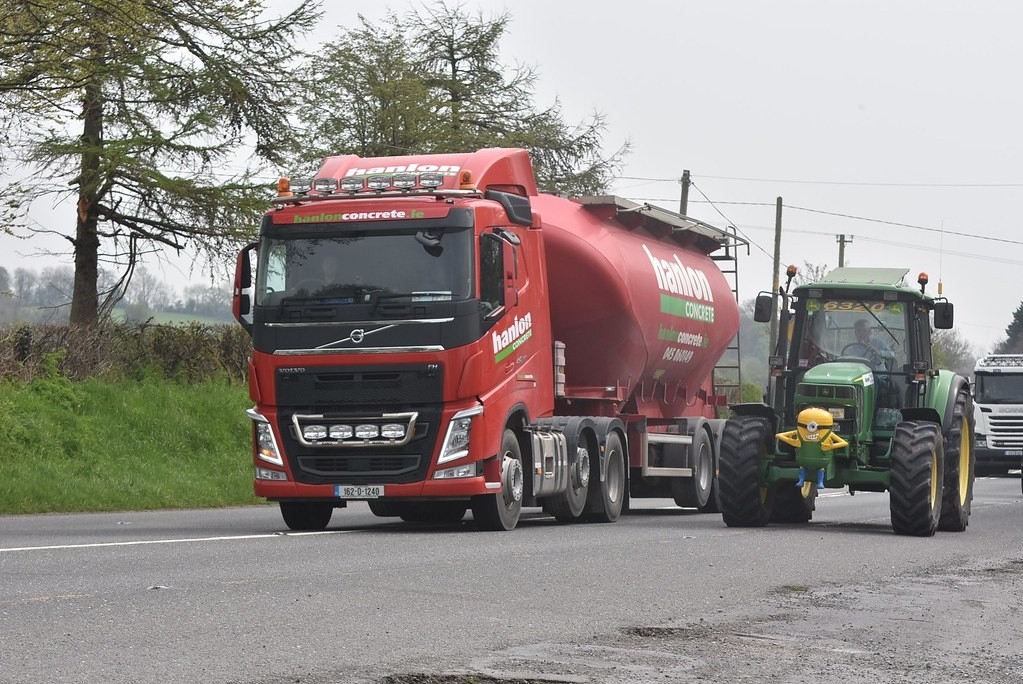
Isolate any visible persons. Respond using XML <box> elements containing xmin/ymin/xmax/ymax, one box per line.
<box><xmin>314</xmin><ymin>254</ymin><xmax>340</xmax><ymax>285</ymax></box>
<box><xmin>849</xmin><ymin>320</ymin><xmax>895</xmax><ymax>408</ymax></box>
<box><xmin>984</xmin><ymin>380</ymin><xmax>1002</xmax><ymax>401</ymax></box>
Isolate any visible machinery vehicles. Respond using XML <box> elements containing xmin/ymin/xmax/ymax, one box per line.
<box><xmin>717</xmin><ymin>263</ymin><xmax>979</xmax><ymax>537</ymax></box>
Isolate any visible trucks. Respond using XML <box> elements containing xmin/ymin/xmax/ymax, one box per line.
<box><xmin>235</xmin><ymin>150</ymin><xmax>755</xmax><ymax>540</ymax></box>
<box><xmin>963</xmin><ymin>353</ymin><xmax>1023</xmax><ymax>482</ymax></box>
